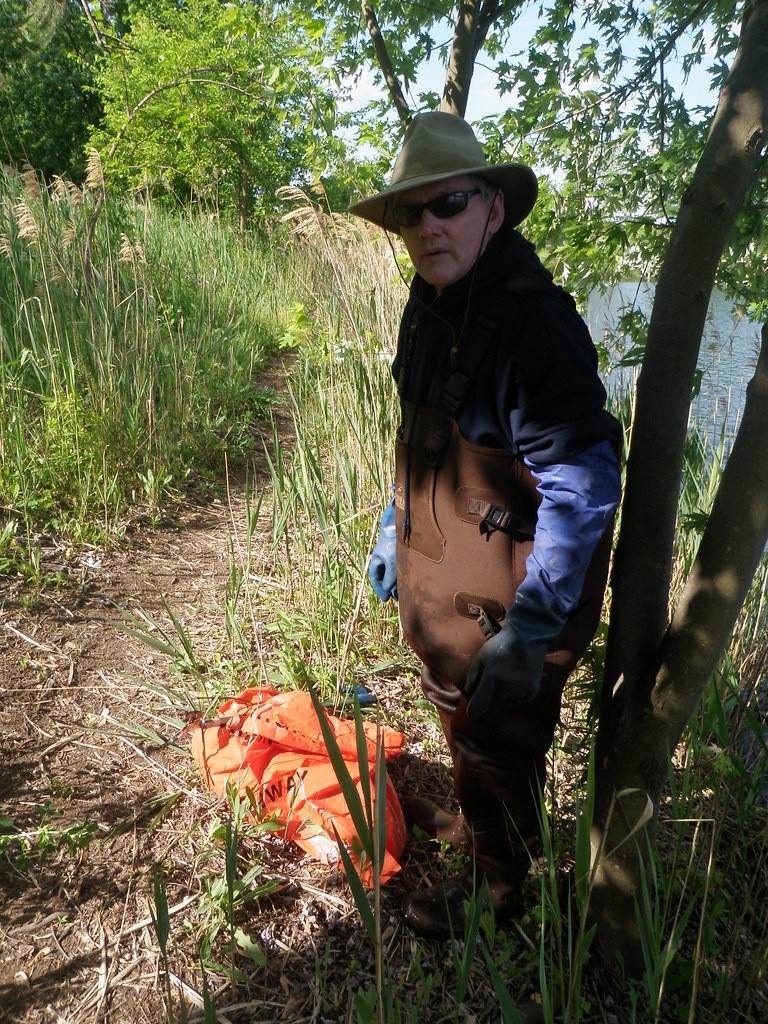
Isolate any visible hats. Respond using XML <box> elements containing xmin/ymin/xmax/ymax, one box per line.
<box><xmin>348</xmin><ymin>112</ymin><xmax>539</xmax><ymax>234</ymax></box>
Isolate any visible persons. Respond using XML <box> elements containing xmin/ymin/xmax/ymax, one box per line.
<box><xmin>345</xmin><ymin>111</ymin><xmax>625</xmax><ymax>940</ymax></box>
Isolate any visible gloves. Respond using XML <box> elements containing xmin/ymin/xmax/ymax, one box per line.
<box><xmin>367</xmin><ymin>494</ymin><xmax>397</xmax><ymax>602</ymax></box>
<box><xmin>465</xmin><ymin>439</ymin><xmax>622</xmax><ymax>718</ymax></box>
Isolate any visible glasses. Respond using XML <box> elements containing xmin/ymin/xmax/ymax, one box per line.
<box><xmin>391</xmin><ymin>187</ymin><xmax>482</xmax><ymax>227</ymax></box>
